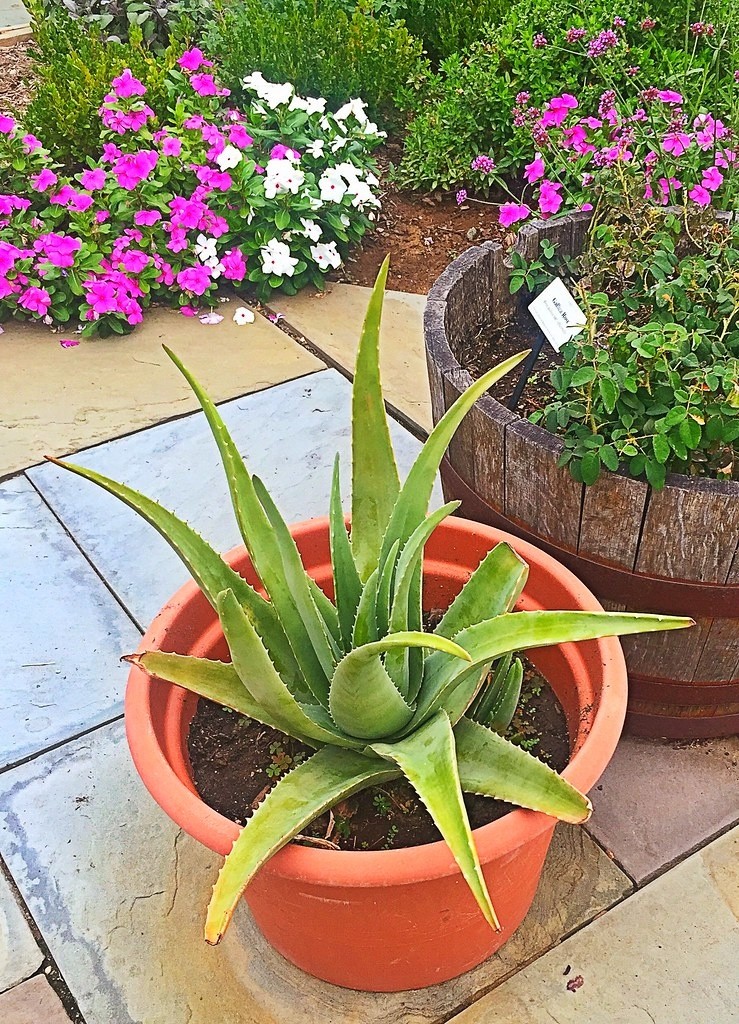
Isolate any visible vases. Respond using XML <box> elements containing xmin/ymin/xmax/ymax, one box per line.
<box><xmin>423</xmin><ymin>216</ymin><xmax>738</xmax><ymax>743</ymax></box>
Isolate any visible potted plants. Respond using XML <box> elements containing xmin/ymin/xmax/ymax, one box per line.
<box><xmin>42</xmin><ymin>249</ymin><xmax>696</xmax><ymax>996</ymax></box>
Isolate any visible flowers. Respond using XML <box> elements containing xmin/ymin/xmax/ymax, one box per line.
<box><xmin>451</xmin><ymin>5</ymin><xmax>738</xmax><ymax>490</ymax></box>
